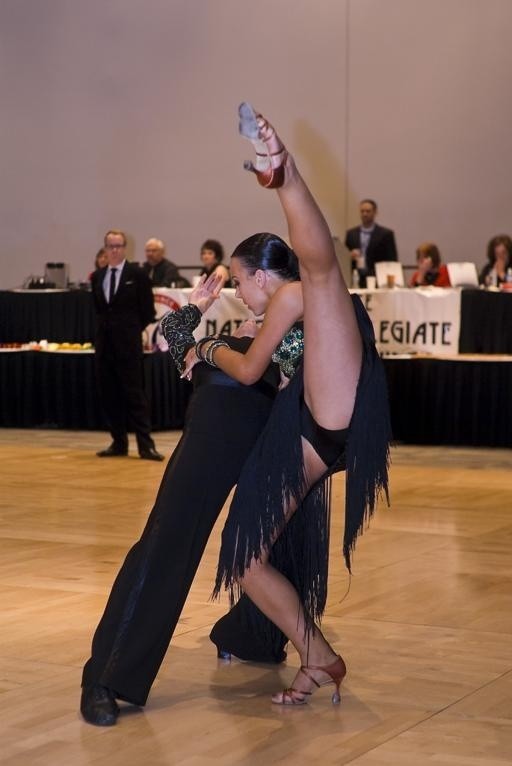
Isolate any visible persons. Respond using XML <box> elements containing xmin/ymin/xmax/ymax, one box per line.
<box><xmin>196</xmin><ymin>236</ymin><xmax>233</xmax><ymax>289</ymax></box>
<box><xmin>475</xmin><ymin>231</ymin><xmax>511</xmax><ymax>286</ymax></box>
<box><xmin>79</xmin><ymin>267</ymin><xmax>319</xmax><ymax>727</ymax></box>
<box><xmin>90</xmin><ymin>229</ymin><xmax>167</xmax><ymax>463</ymax></box>
<box><xmin>340</xmin><ymin>200</ymin><xmax>399</xmax><ymax>287</ymax></box>
<box><xmin>88</xmin><ymin>247</ymin><xmax>110</xmax><ymax>281</ymax></box>
<box><xmin>179</xmin><ymin>97</ymin><xmax>396</xmax><ymax>709</ymax></box>
<box><xmin>408</xmin><ymin>243</ymin><xmax>453</xmax><ymax>285</ymax></box>
<box><xmin>140</xmin><ymin>237</ymin><xmax>179</xmax><ymax>285</ymax></box>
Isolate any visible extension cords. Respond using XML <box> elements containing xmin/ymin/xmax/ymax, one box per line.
<box><xmin>28</xmin><ymin>281</ymin><xmax>56</xmax><ymax>289</ymax></box>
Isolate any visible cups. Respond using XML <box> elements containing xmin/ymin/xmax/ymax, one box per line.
<box><xmin>366</xmin><ymin>276</ymin><xmax>376</xmax><ymax>290</ymax></box>
<box><xmin>192</xmin><ymin>275</ymin><xmax>201</xmax><ymax>287</ymax></box>
<box><xmin>386</xmin><ymin>274</ymin><xmax>394</xmax><ymax>288</ymax></box>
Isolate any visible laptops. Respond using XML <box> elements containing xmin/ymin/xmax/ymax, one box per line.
<box><xmin>373</xmin><ymin>260</ymin><xmax>405</xmax><ymax>288</ymax></box>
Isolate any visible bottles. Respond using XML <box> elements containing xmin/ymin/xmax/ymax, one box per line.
<box><xmin>352</xmin><ymin>269</ymin><xmax>358</xmax><ymax>288</ymax></box>
<box><xmin>507</xmin><ymin>267</ymin><xmax>512</xmax><ymax>292</ymax></box>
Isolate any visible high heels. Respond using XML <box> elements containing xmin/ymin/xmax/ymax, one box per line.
<box><xmin>239</xmin><ymin>103</ymin><xmax>289</xmax><ymax>190</ymax></box>
<box><xmin>271</xmin><ymin>654</ymin><xmax>346</xmax><ymax>706</ymax></box>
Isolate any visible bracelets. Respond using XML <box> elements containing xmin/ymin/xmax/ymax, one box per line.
<box><xmin>195</xmin><ymin>335</ymin><xmax>231</xmax><ymax>367</ymax></box>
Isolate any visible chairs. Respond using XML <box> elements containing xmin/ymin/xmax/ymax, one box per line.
<box><xmin>372</xmin><ymin>263</ymin><xmax>404</xmax><ymax>287</ymax></box>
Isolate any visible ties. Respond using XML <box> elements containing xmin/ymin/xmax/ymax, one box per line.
<box><xmin>108</xmin><ymin>267</ymin><xmax>117</xmax><ymax>303</ymax></box>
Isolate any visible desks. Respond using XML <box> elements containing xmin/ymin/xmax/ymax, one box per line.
<box><xmin>0</xmin><ymin>283</ymin><xmax>512</xmax><ymax>442</ymax></box>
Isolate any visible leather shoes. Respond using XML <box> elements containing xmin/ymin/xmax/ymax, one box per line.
<box><xmin>139</xmin><ymin>448</ymin><xmax>165</xmax><ymax>460</ymax></box>
<box><xmin>96</xmin><ymin>447</ymin><xmax>128</xmax><ymax>457</ymax></box>
<box><xmin>80</xmin><ymin>685</ymin><xmax>119</xmax><ymax>726</ymax></box>
